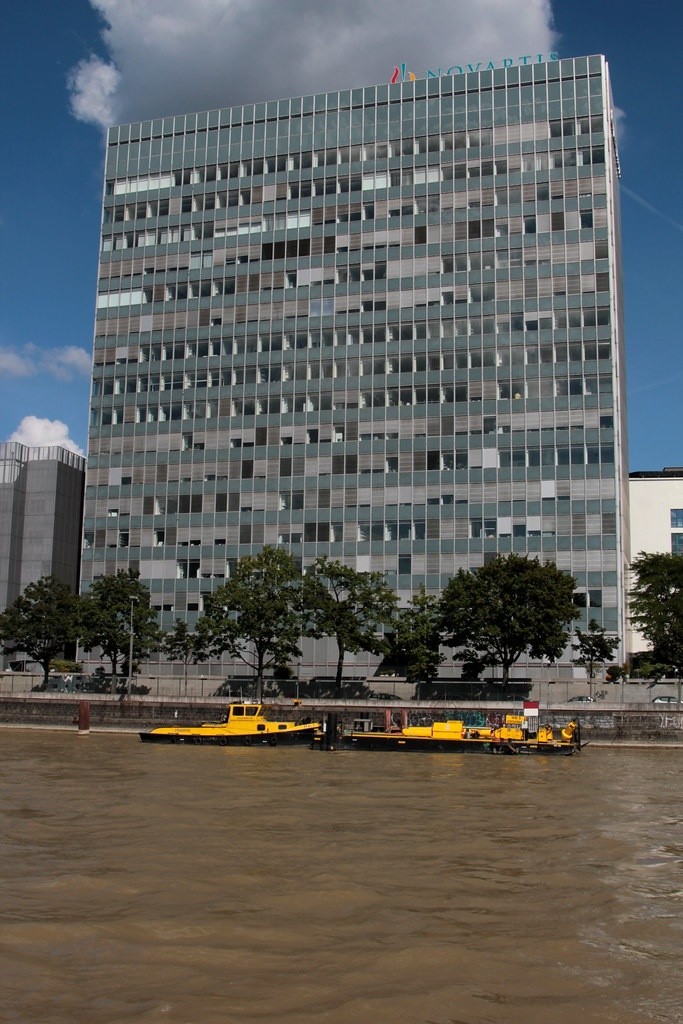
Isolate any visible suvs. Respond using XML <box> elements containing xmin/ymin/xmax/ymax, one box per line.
<box><xmin>653</xmin><ymin>695</ymin><xmax>683</xmax><ymax>704</ymax></box>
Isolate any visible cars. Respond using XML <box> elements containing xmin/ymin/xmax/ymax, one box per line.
<box><xmin>566</xmin><ymin>696</ymin><xmax>595</xmax><ymax>703</ymax></box>
<box><xmin>440</xmin><ymin>695</ymin><xmax>469</xmax><ymax>701</ymax></box>
<box><xmin>367</xmin><ymin>694</ymin><xmax>405</xmax><ymax>701</ymax></box>
<box><xmin>504</xmin><ymin>695</ymin><xmax>531</xmax><ymax>702</ymax></box>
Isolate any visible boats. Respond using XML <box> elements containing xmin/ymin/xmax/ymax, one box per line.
<box><xmin>138</xmin><ymin>703</ymin><xmax>321</xmax><ymax>746</ymax></box>
<box><xmin>334</xmin><ymin>710</ymin><xmax>593</xmax><ymax>757</ymax></box>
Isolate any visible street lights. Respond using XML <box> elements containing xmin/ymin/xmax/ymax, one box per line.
<box><xmin>127</xmin><ymin>596</ymin><xmax>139</xmax><ymax>696</ymax></box>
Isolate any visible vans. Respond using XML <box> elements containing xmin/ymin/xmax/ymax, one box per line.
<box><xmin>49</xmin><ymin>676</ymin><xmax>95</xmax><ymax>694</ymax></box>
